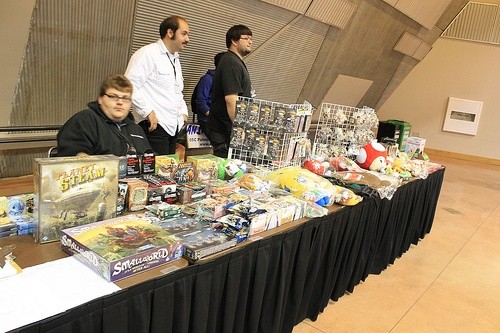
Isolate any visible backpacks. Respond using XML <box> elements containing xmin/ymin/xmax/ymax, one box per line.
<box><xmin>191</xmin><ymin>73</ymin><xmax>216</xmax><ymax>114</ymax></box>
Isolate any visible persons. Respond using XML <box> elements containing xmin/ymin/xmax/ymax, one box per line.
<box><xmin>56</xmin><ymin>74</ymin><xmax>153</xmax><ymax>157</ymax></box>
<box><xmin>190</xmin><ymin>50</ymin><xmax>228</xmax><ymax>158</ymax></box>
<box><xmin>123</xmin><ymin>15</ymin><xmax>191</xmax><ymax>156</ymax></box>
<box><xmin>208</xmin><ymin>25</ymin><xmax>253</xmax><ymax>150</ymax></box>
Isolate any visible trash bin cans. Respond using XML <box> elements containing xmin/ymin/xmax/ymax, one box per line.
<box><xmin>387</xmin><ymin>119</ymin><xmax>412</xmax><ymax>153</ymax></box>
<box><xmin>376</xmin><ymin>120</ymin><xmax>401</xmax><ymax>147</ymax></box>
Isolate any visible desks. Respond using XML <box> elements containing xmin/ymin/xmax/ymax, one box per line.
<box><xmin>0</xmin><ymin>162</ymin><xmax>446</xmax><ymax>333</ymax></box>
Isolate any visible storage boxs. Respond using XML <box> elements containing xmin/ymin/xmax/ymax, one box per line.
<box><xmin>405</xmin><ymin>136</ymin><xmax>426</xmax><ymax>154</ymax></box>
<box><xmin>226</xmin><ymin>199</ymin><xmax>280</xmax><ymax>235</ymax></box>
<box><xmin>176</xmin><ymin>181</ymin><xmax>210</xmax><ymax>204</ymax></box>
<box><xmin>151</xmin><ymin>215</ymin><xmax>237</xmax><ymax>261</ymax></box>
<box><xmin>32</xmin><ymin>154</ymin><xmax>119</xmax><ymax>244</ymax></box>
<box><xmin>187</xmin><ymin>154</ymin><xmax>225</xmax><ymax>181</ymax></box>
<box><xmin>251</xmin><ymin>190</ymin><xmax>306</xmax><ymax>224</ymax></box>
<box><xmin>61</xmin><ymin>214</ymin><xmax>183</xmax><ymax>283</ymax></box>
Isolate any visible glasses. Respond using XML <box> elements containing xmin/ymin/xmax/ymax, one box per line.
<box><xmin>103</xmin><ymin>92</ymin><xmax>131</xmax><ymax>102</ymax></box>
<box><xmin>240</xmin><ymin>36</ymin><xmax>251</xmax><ymax>41</ymax></box>
